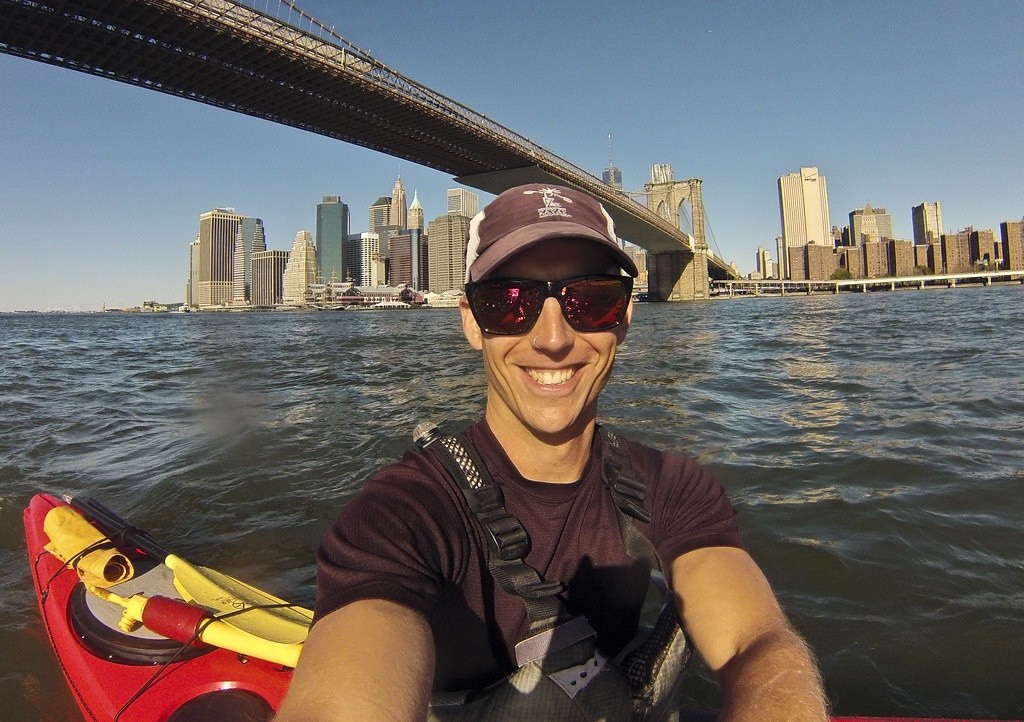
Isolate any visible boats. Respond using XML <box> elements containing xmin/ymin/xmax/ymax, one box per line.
<box><xmin>22</xmin><ymin>494</ymin><xmax>433</xmax><ymax>722</ymax></box>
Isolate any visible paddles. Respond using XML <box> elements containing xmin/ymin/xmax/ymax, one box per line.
<box><xmin>59</xmin><ymin>490</ymin><xmax>317</xmax><ymax>648</ymax></box>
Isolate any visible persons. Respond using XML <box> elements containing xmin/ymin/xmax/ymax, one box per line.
<box><xmin>270</xmin><ymin>183</ymin><xmax>826</xmax><ymax>722</ymax></box>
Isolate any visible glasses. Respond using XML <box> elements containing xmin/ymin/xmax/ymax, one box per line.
<box><xmin>464</xmin><ymin>274</ymin><xmax>633</xmax><ymax>335</ymax></box>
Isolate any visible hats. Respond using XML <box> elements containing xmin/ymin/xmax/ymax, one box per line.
<box><xmin>465</xmin><ymin>183</ymin><xmax>639</xmax><ymax>288</ymax></box>
